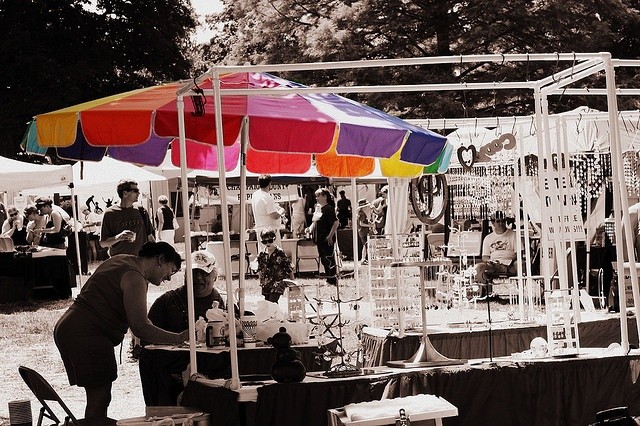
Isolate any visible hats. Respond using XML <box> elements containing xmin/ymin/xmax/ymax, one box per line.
<box><xmin>358</xmin><ymin>198</ymin><xmax>369</xmax><ymax>208</ymax></box>
<box><xmin>80</xmin><ymin>205</ymin><xmax>88</xmax><ymax>212</ymax></box>
<box><xmin>184</xmin><ymin>250</ymin><xmax>216</xmax><ymax>273</ymax></box>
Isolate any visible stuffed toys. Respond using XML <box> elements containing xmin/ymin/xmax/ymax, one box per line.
<box><xmin>266</xmin><ymin>326</ymin><xmax>307</xmax><ymax>388</ymax></box>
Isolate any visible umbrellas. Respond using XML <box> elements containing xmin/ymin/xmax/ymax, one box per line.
<box><xmin>21</xmin><ymin>69</ymin><xmax>449</xmax><ymax>314</ymax></box>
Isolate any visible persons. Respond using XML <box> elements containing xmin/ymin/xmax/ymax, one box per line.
<box><xmin>467</xmin><ymin>211</ymin><xmax>527</xmax><ymax>295</ymax></box>
<box><xmin>356</xmin><ymin>197</ymin><xmax>375</xmax><ymax>265</ymax></box>
<box><xmin>147</xmin><ymin>250</ymin><xmax>226</xmax><ymax>333</ymax></box>
<box><xmin>99</xmin><ymin>180</ymin><xmax>156</xmax><ymax>257</ymax></box>
<box><xmin>84</xmin><ymin>221</ymin><xmax>101</xmax><ymax>229</ymax></box>
<box><xmin>85</xmin><ymin>195</ymin><xmax>95</xmax><ymax>212</ymax></box>
<box><xmin>53</xmin><ymin>240</ymin><xmax>206</xmax><ymax>417</ymax></box>
<box><xmin>335</xmin><ymin>190</ymin><xmax>352</xmax><ymax>228</ymax></box>
<box><xmin>0</xmin><ymin>190</ymin><xmax>87</xmax><ymax>276</ymax></box>
<box><xmin>463</xmin><ymin>219</ymin><xmax>482</xmax><ymax>232</ymax></box>
<box><xmin>248</xmin><ymin>228</ymin><xmax>296</xmax><ymax>303</ymax></box>
<box><xmin>304</xmin><ymin>187</ymin><xmax>340</xmax><ymax>287</ymax></box>
<box><xmin>368</xmin><ymin>185</ymin><xmax>389</xmax><ymax>217</ymax></box>
<box><xmin>155</xmin><ymin>194</ymin><xmax>175</xmax><ymax>243</ymax></box>
<box><xmin>618</xmin><ymin>201</ymin><xmax>640</xmax><ymax>263</ymax></box>
<box><xmin>291</xmin><ymin>193</ymin><xmax>308</xmax><ymax>239</ymax></box>
<box><xmin>101</xmin><ymin>197</ymin><xmax>113</xmax><ymax>208</ymax></box>
<box><xmin>94</xmin><ymin>202</ymin><xmax>104</xmax><ymax>213</ymax></box>
<box><xmin>251</xmin><ymin>174</ymin><xmax>285</xmax><ymax>251</ymax></box>
<box><xmin>370</xmin><ymin>191</ymin><xmax>385</xmax><ymax>238</ymax></box>
<box><xmin>210</xmin><ymin>212</ymin><xmax>224</xmax><ymax>241</ymax></box>
<box><xmin>489</xmin><ymin>213</ymin><xmax>496</xmax><ymax>225</ymax></box>
<box><xmin>87</xmin><ymin>232</ymin><xmax>98</xmax><ymax>265</ymax></box>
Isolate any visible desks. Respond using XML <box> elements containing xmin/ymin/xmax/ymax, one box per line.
<box><xmin>358</xmin><ymin>310</ymin><xmax>640</xmax><ymax>369</ymax></box>
<box><xmin>206</xmin><ymin>237</ymin><xmax>320</xmax><ymax>277</ymax></box>
<box><xmin>187</xmin><ymin>348</ymin><xmax>638</xmax><ymax>425</ymax></box>
<box><xmin>0</xmin><ymin>242</ymin><xmax>68</xmax><ymax>304</ymax></box>
<box><xmin>139</xmin><ymin>336</ymin><xmax>337</xmax><ymax>418</ymax></box>
<box><xmin>189</xmin><ymin>227</ymin><xmax>293</xmax><ymax>249</ymax></box>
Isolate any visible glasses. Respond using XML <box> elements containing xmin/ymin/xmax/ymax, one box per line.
<box><xmin>133</xmin><ymin>188</ymin><xmax>140</xmax><ymax>192</ymax></box>
<box><xmin>261</xmin><ymin>236</ymin><xmax>275</xmax><ymax>243</ymax></box>
<box><xmin>10</xmin><ymin>210</ymin><xmax>19</xmax><ymax>216</ymax></box>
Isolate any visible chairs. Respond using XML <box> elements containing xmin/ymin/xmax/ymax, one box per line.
<box><xmin>296</xmin><ymin>238</ymin><xmax>319</xmax><ymax>278</ymax></box>
<box><xmin>284</xmin><ymin>279</ymin><xmax>347</xmax><ymax>354</ymax></box>
<box><xmin>19</xmin><ymin>365</ymin><xmax>116</xmax><ymax>426</ymax></box>
<box><xmin>223</xmin><ymin>241</ymin><xmax>251</xmax><ymax>280</ymax></box>
